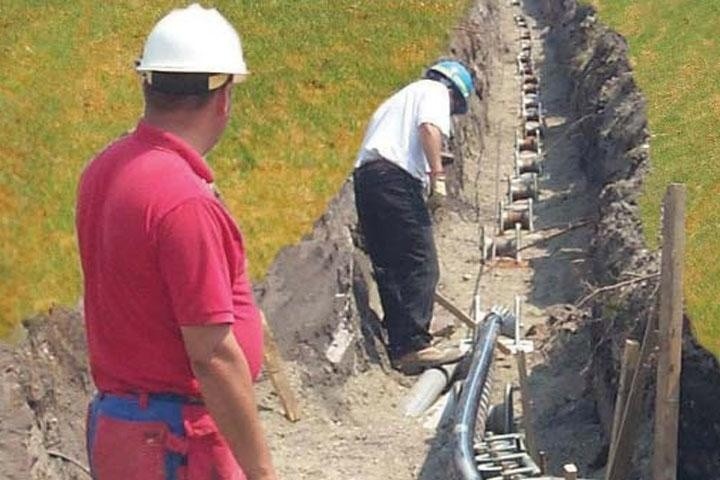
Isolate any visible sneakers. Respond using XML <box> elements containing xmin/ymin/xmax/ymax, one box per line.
<box><xmin>402</xmin><ymin>343</ymin><xmax>466</xmax><ymax>375</ymax></box>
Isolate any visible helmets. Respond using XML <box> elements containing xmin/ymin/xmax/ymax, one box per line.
<box><xmin>427</xmin><ymin>61</ymin><xmax>473</xmax><ymax>114</ymax></box>
<box><xmin>135</xmin><ymin>3</ymin><xmax>251</xmax><ymax>76</ymax></box>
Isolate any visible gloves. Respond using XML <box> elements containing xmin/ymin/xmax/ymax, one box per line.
<box><xmin>429</xmin><ymin>175</ymin><xmax>447</xmax><ymax>199</ymax></box>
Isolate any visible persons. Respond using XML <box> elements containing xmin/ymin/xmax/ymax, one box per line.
<box><xmin>76</xmin><ymin>3</ymin><xmax>277</xmax><ymax>480</ymax></box>
<box><xmin>352</xmin><ymin>60</ymin><xmax>473</xmax><ymax>375</ymax></box>
